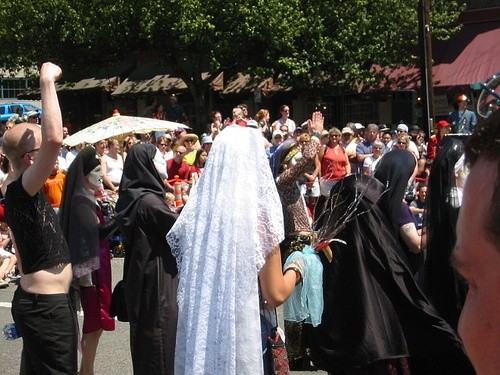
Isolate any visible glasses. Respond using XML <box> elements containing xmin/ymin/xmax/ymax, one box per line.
<box><xmin>185</xmin><ymin>139</ymin><xmax>194</xmax><ymax>142</ymax></box>
<box><xmin>398</xmin><ymin>141</ymin><xmax>407</xmax><ymax>145</ymax></box>
<box><xmin>476</xmin><ymin>71</ymin><xmax>500</xmax><ymax>120</ymax></box>
<box><xmin>331</xmin><ymin>134</ymin><xmax>338</xmax><ymax>138</ymax></box>
<box><xmin>397</xmin><ymin>130</ymin><xmax>406</xmax><ymax>132</ymax></box>
<box><xmin>159</xmin><ymin>143</ymin><xmax>168</xmax><ymax>147</ymax></box>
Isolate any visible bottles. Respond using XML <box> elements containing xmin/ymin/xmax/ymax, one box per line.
<box><xmin>2</xmin><ymin>323</ymin><xmax>21</xmax><ymax>341</ymax></box>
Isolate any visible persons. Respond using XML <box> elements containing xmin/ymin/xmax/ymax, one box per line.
<box><xmin>0</xmin><ymin>62</ymin><xmax>500</xmax><ymax>375</ymax></box>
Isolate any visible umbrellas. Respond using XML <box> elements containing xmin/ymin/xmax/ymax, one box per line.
<box><xmin>62</xmin><ymin>109</ymin><xmax>192</xmax><ymax>165</ymax></box>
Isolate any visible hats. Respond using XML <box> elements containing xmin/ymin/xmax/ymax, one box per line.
<box><xmin>378</xmin><ymin>123</ymin><xmax>390</xmax><ymax>132</ymax></box>
<box><xmin>436</xmin><ymin>120</ymin><xmax>455</xmax><ymax>128</ymax></box>
<box><xmin>409</xmin><ymin>125</ymin><xmax>421</xmax><ymax>131</ymax></box>
<box><xmin>200</xmin><ymin>135</ymin><xmax>213</xmax><ymax>148</ymax></box>
<box><xmin>341</xmin><ymin>127</ymin><xmax>354</xmax><ymax>135</ymax></box>
<box><xmin>178</xmin><ymin>134</ymin><xmax>199</xmax><ymax>146</ymax></box>
<box><xmin>457</xmin><ymin>94</ymin><xmax>470</xmax><ymax>102</ymax></box>
<box><xmin>294</xmin><ymin>126</ymin><xmax>303</xmax><ymax>133</ymax></box>
<box><xmin>355</xmin><ymin>123</ymin><xmax>365</xmax><ymax>129</ymax></box>
<box><xmin>397</xmin><ymin>124</ymin><xmax>409</xmax><ymax>133</ymax></box>
<box><xmin>247</xmin><ymin>120</ymin><xmax>259</xmax><ymax>128</ymax></box>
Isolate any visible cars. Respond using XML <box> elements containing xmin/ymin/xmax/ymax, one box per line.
<box><xmin>0</xmin><ymin>101</ymin><xmax>42</xmax><ymax>124</ymax></box>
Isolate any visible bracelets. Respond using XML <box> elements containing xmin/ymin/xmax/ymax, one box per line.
<box><xmin>283</xmin><ymin>267</ymin><xmax>302</xmax><ymax>285</ymax></box>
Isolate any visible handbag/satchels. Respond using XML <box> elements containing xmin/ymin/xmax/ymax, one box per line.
<box><xmin>267</xmin><ymin>325</ymin><xmax>291</xmax><ymax>375</ymax></box>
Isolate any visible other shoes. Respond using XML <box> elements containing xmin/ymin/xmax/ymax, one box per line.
<box><xmin>0</xmin><ymin>274</ymin><xmax>21</xmax><ymax>287</ymax></box>
